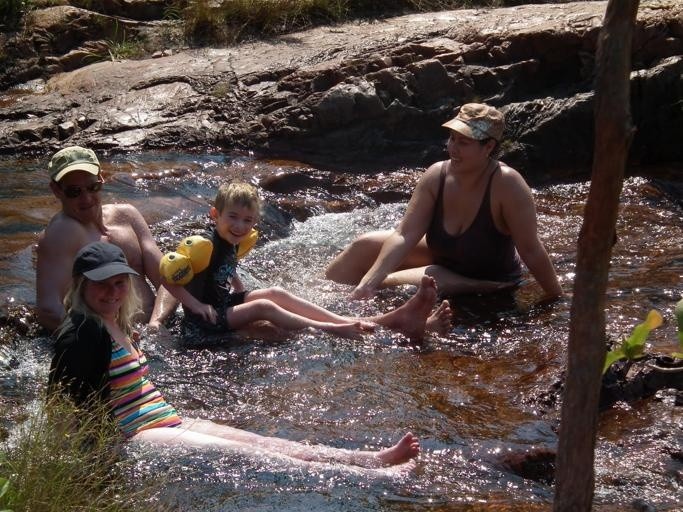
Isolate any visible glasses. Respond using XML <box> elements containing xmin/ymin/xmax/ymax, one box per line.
<box><xmin>52</xmin><ymin>173</ymin><xmax>106</xmax><ymax>199</ymax></box>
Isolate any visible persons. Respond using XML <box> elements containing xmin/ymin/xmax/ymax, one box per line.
<box><xmin>159</xmin><ymin>180</ymin><xmax>378</xmax><ymax>339</ymax></box>
<box><xmin>35</xmin><ymin>145</ymin><xmax>179</xmax><ymax>335</ymax></box>
<box><xmin>322</xmin><ymin>103</ymin><xmax>565</xmax><ymax>303</ymax></box>
<box><xmin>48</xmin><ymin>243</ymin><xmax>421</xmax><ymax>478</ymax></box>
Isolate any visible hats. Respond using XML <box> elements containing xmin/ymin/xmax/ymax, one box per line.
<box><xmin>47</xmin><ymin>145</ymin><xmax>102</xmax><ymax>184</ymax></box>
<box><xmin>72</xmin><ymin>241</ymin><xmax>141</xmax><ymax>287</ymax></box>
<box><xmin>440</xmin><ymin>101</ymin><xmax>507</xmax><ymax>143</ymax></box>
